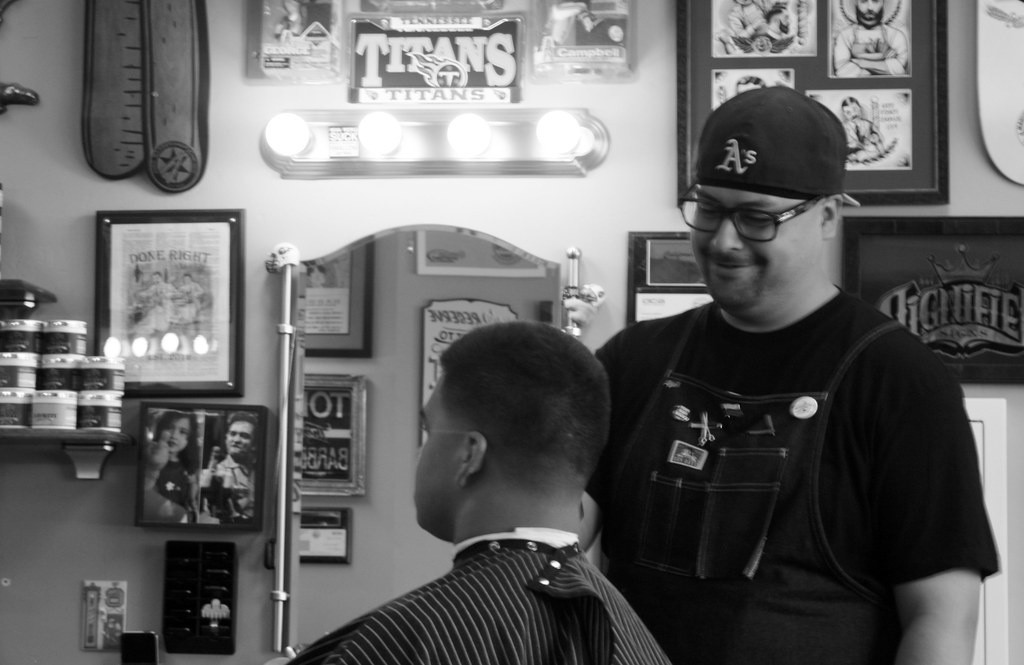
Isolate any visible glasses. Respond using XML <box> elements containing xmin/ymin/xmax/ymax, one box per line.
<box><xmin>677</xmin><ymin>185</ymin><xmax>822</xmax><ymax>241</ymax></box>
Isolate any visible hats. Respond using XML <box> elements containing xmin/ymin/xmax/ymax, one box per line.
<box><xmin>695</xmin><ymin>85</ymin><xmax>863</xmax><ymax>209</ymax></box>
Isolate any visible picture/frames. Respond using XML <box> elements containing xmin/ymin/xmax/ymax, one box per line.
<box><xmin>305</xmin><ymin>241</ymin><xmax>375</xmax><ymax>360</ymax></box>
<box><xmin>298</xmin><ymin>373</ymin><xmax>369</xmax><ymax>496</ymax></box>
<box><xmin>677</xmin><ymin>0</ymin><xmax>950</xmax><ymax>208</ymax></box>
<box><xmin>134</xmin><ymin>401</ymin><xmax>269</xmax><ymax>534</ymax></box>
<box><xmin>842</xmin><ymin>215</ymin><xmax>1024</xmax><ymax>386</ymax></box>
<box><xmin>94</xmin><ymin>208</ymin><xmax>246</xmax><ymax>399</ymax></box>
<box><xmin>412</xmin><ymin>230</ymin><xmax>548</xmax><ymax>279</ymax></box>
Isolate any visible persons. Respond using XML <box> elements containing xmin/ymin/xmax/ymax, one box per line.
<box><xmin>199</xmin><ymin>414</ymin><xmax>256</xmax><ymax>525</ymax></box>
<box><xmin>145</xmin><ymin>408</ymin><xmax>199</xmax><ymax>524</ymax></box>
<box><xmin>592</xmin><ymin>86</ymin><xmax>1001</xmax><ymax>665</ymax></box>
<box><xmin>265</xmin><ymin>321</ymin><xmax>671</xmax><ymax>665</ymax></box>
